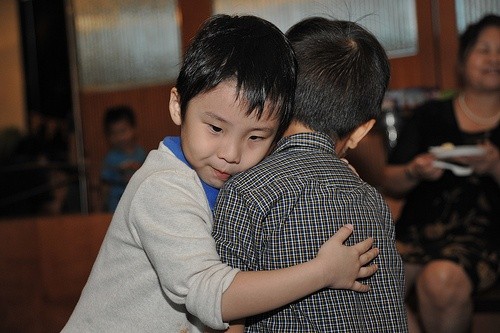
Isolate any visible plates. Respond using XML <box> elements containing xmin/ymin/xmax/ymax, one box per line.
<box><xmin>430</xmin><ymin>145</ymin><xmax>486</xmax><ymax>158</ymax></box>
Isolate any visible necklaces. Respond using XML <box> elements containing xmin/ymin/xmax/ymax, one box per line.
<box><xmin>458</xmin><ymin>93</ymin><xmax>500</xmax><ymax>125</ymax></box>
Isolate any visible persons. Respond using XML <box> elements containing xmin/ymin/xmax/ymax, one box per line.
<box><xmin>211</xmin><ymin>16</ymin><xmax>410</xmax><ymax>333</ymax></box>
<box><xmin>100</xmin><ymin>104</ymin><xmax>149</xmax><ymax>213</ymax></box>
<box><xmin>59</xmin><ymin>13</ymin><xmax>380</xmax><ymax>333</ymax></box>
<box><xmin>382</xmin><ymin>13</ymin><xmax>500</xmax><ymax>333</ymax></box>
